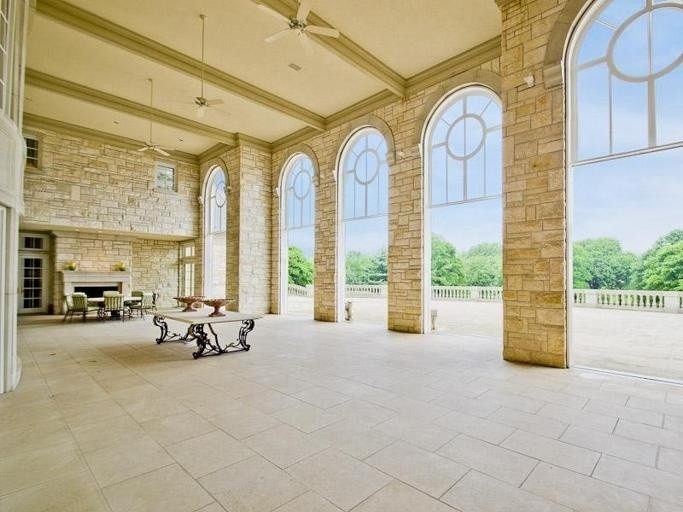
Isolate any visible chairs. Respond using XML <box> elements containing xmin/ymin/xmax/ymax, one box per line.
<box><xmin>61</xmin><ymin>291</ymin><xmax>144</xmax><ymax>325</ymax></box>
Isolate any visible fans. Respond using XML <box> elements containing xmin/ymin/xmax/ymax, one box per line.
<box><xmin>134</xmin><ymin>14</ymin><xmax>230</xmax><ymax>157</ymax></box>
<box><xmin>257</xmin><ymin>1</ymin><xmax>339</xmax><ymax>58</ymax></box>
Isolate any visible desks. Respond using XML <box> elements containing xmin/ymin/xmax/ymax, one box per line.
<box><xmin>144</xmin><ymin>309</ymin><xmax>263</xmax><ymax>359</ymax></box>
